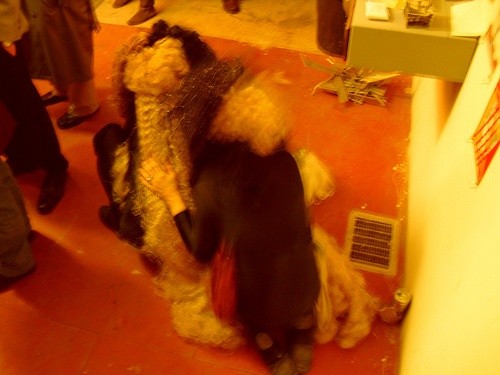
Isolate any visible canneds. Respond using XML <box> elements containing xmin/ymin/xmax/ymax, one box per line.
<box><xmin>391</xmin><ymin>288</ymin><xmax>411</xmax><ymax>316</ymax></box>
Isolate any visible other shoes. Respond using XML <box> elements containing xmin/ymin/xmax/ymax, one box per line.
<box><xmin>98</xmin><ymin>207</ymin><xmax>144</xmax><ymax>247</ymax></box>
<box><xmin>252</xmin><ymin>332</ymin><xmax>299</xmax><ymax>375</ymax></box>
<box><xmin>0</xmin><ymin>263</ymin><xmax>36</xmax><ymax>291</ymax></box>
<box><xmin>293</xmin><ymin>311</ymin><xmax>316</xmax><ymax>372</ymax></box>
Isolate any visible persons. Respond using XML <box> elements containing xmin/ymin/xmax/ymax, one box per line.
<box><xmin>19</xmin><ymin>0</ymin><xmax>99</xmax><ymax>129</ymax></box>
<box><xmin>93</xmin><ymin>36</ymin><xmax>198</xmax><ymax>264</ymax></box>
<box><xmin>0</xmin><ymin>0</ymin><xmax>68</xmax><ymax>214</ymax></box>
<box><xmin>0</xmin><ymin>156</ymin><xmax>36</xmax><ymax>290</ymax></box>
<box><xmin>113</xmin><ymin>0</ymin><xmax>155</xmax><ymax>25</ymax></box>
<box><xmin>136</xmin><ymin>80</ymin><xmax>320</xmax><ymax>375</ymax></box>
<box><xmin>317</xmin><ymin>0</ymin><xmax>348</xmax><ymax>58</ymax></box>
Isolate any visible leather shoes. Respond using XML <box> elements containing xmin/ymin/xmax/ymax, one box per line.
<box><xmin>57</xmin><ymin>107</ymin><xmax>101</xmax><ymax>128</ymax></box>
<box><xmin>39</xmin><ymin>172</ymin><xmax>66</xmax><ymax>214</ymax></box>
<box><xmin>6</xmin><ymin>156</ymin><xmax>37</xmax><ymax>175</ymax></box>
<box><xmin>40</xmin><ymin>91</ymin><xmax>70</xmax><ymax>104</ymax></box>
<box><xmin>129</xmin><ymin>9</ymin><xmax>159</xmax><ymax>25</ymax></box>
<box><xmin>225</xmin><ymin>0</ymin><xmax>240</xmax><ymax>14</ymax></box>
<box><xmin>113</xmin><ymin>0</ymin><xmax>132</xmax><ymax>7</ymax></box>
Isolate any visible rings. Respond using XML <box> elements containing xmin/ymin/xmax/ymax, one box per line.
<box><xmin>146</xmin><ymin>176</ymin><xmax>152</xmax><ymax>182</ymax></box>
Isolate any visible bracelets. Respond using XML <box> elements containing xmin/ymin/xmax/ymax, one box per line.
<box><xmin>3</xmin><ymin>42</ymin><xmax>12</xmax><ymax>47</ymax></box>
